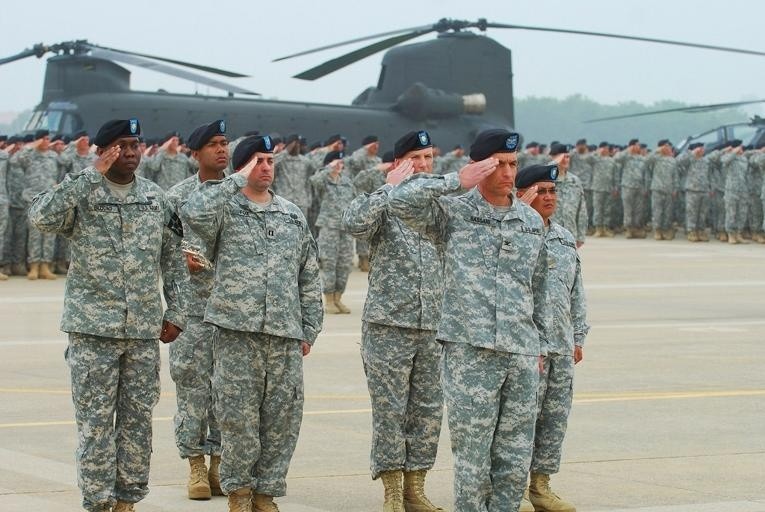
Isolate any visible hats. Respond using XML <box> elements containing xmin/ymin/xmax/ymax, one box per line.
<box><xmin>469</xmin><ymin>129</ymin><xmax>519</xmax><ymax>162</ymax></box>
<box><xmin>232</xmin><ymin>136</ymin><xmax>275</xmax><ymax>171</ymax></box>
<box><xmin>526</xmin><ymin>139</ymin><xmax>765</xmax><ymax>156</ymax></box>
<box><xmin>0</xmin><ymin>129</ymin><xmax>187</xmax><ymax>146</ymax></box>
<box><xmin>187</xmin><ymin>119</ymin><xmax>227</xmax><ymax>151</ymax></box>
<box><xmin>514</xmin><ymin>164</ymin><xmax>559</xmax><ymax>188</ymax></box>
<box><xmin>95</xmin><ymin>119</ymin><xmax>141</xmax><ymax>146</ymax></box>
<box><xmin>272</xmin><ymin>130</ymin><xmax>465</xmax><ymax>166</ymax></box>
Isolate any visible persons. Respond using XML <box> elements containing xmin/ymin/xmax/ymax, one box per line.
<box><xmin>341</xmin><ymin>130</ymin><xmax>445</xmax><ymax>512</ymax></box>
<box><xmin>165</xmin><ymin>120</ymin><xmax>229</xmax><ymax>498</ymax></box>
<box><xmin>135</xmin><ymin>132</ymin><xmax>199</xmax><ymax>190</ymax></box>
<box><xmin>514</xmin><ymin>165</ymin><xmax>590</xmax><ymax>512</ymax></box>
<box><xmin>272</xmin><ymin>133</ymin><xmax>396</xmax><ymax>314</ymax></box>
<box><xmin>516</xmin><ymin>134</ymin><xmax>765</xmax><ymax>249</ymax></box>
<box><xmin>386</xmin><ymin>129</ymin><xmax>553</xmax><ymax>512</ymax></box>
<box><xmin>0</xmin><ymin>132</ymin><xmax>99</xmax><ymax>280</ymax></box>
<box><xmin>27</xmin><ymin>118</ymin><xmax>191</xmax><ymax>512</ymax></box>
<box><xmin>223</xmin><ymin>130</ymin><xmax>258</xmax><ymax>177</ymax></box>
<box><xmin>180</xmin><ymin>137</ymin><xmax>324</xmax><ymax>512</ymax></box>
<box><xmin>433</xmin><ymin>142</ymin><xmax>470</xmax><ymax>174</ymax></box>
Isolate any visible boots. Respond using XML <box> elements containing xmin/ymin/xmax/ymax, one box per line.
<box><xmin>403</xmin><ymin>470</ymin><xmax>443</xmax><ymax>512</ymax></box>
<box><xmin>586</xmin><ymin>220</ymin><xmax>765</xmax><ymax>244</ymax></box>
<box><xmin>208</xmin><ymin>455</ymin><xmax>224</xmax><ymax>496</ymax></box>
<box><xmin>334</xmin><ymin>291</ymin><xmax>350</xmax><ymax>313</ymax></box>
<box><xmin>186</xmin><ymin>454</ymin><xmax>211</xmax><ymax>499</ymax></box>
<box><xmin>252</xmin><ymin>488</ymin><xmax>280</xmax><ymax>512</ymax></box>
<box><xmin>324</xmin><ymin>293</ymin><xmax>341</xmax><ymax>313</ymax></box>
<box><xmin>360</xmin><ymin>256</ymin><xmax>369</xmax><ymax>272</ymax></box>
<box><xmin>0</xmin><ymin>258</ymin><xmax>68</xmax><ymax>280</ymax></box>
<box><xmin>380</xmin><ymin>470</ymin><xmax>404</xmax><ymax>512</ymax></box>
<box><xmin>518</xmin><ymin>488</ymin><xmax>536</xmax><ymax>512</ymax></box>
<box><xmin>228</xmin><ymin>487</ymin><xmax>253</xmax><ymax>512</ymax></box>
<box><xmin>528</xmin><ymin>471</ymin><xmax>576</xmax><ymax>512</ymax></box>
